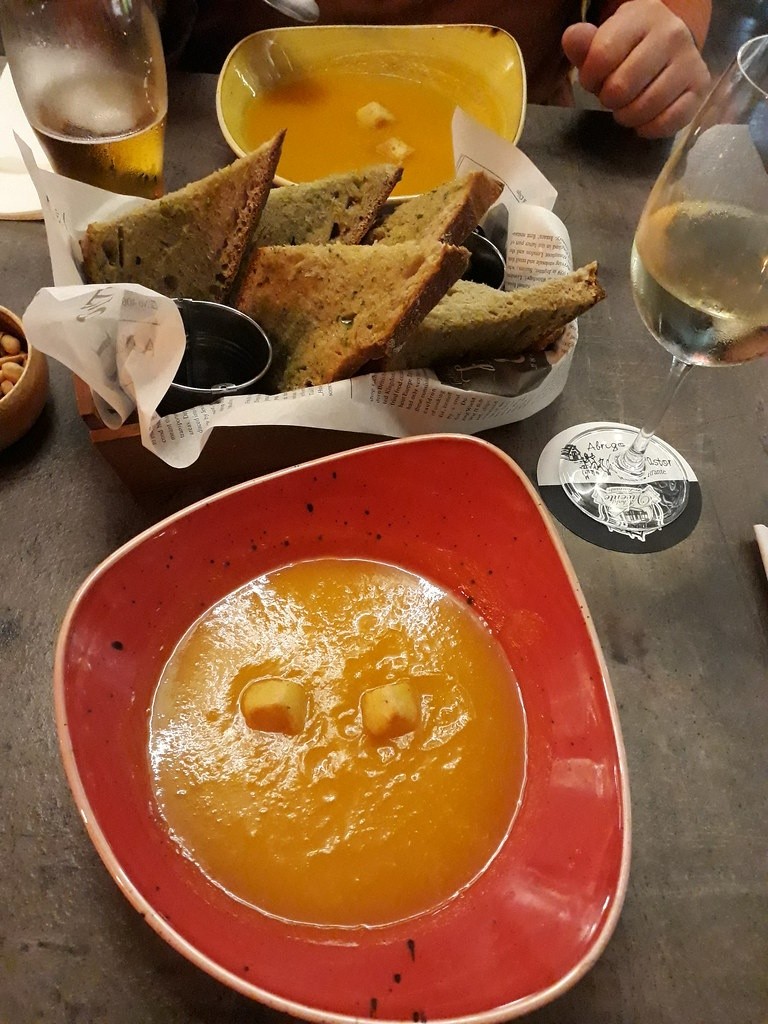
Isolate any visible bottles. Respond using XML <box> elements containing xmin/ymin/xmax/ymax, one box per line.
<box><xmin>0</xmin><ymin>0</ymin><xmax>168</xmax><ymax>201</ymax></box>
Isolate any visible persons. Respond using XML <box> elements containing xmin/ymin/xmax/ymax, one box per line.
<box><xmin>154</xmin><ymin>0</ymin><xmax>712</xmax><ymax>141</ymax></box>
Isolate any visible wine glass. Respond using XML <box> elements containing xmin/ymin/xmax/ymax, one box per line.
<box><xmin>561</xmin><ymin>35</ymin><xmax>768</xmax><ymax>536</ymax></box>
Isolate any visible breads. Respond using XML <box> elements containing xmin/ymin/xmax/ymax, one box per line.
<box><xmin>68</xmin><ymin>128</ymin><xmax>604</xmax><ymax>388</ymax></box>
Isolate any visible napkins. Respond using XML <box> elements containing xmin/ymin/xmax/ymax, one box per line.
<box><xmin>0</xmin><ymin>61</ymin><xmax>54</xmax><ymax>221</ymax></box>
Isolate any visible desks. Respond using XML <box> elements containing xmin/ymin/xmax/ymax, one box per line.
<box><xmin>0</xmin><ymin>57</ymin><xmax>768</xmax><ymax>1024</ymax></box>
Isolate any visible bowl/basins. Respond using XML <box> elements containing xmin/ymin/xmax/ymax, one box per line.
<box><xmin>53</xmin><ymin>433</ymin><xmax>632</xmax><ymax>1024</ymax></box>
<box><xmin>462</xmin><ymin>232</ymin><xmax>507</xmax><ymax>292</ymax></box>
<box><xmin>171</xmin><ymin>296</ymin><xmax>271</xmax><ymax>408</ymax></box>
<box><xmin>0</xmin><ymin>304</ymin><xmax>46</xmax><ymax>451</ymax></box>
<box><xmin>215</xmin><ymin>23</ymin><xmax>526</xmax><ymax>206</ymax></box>
